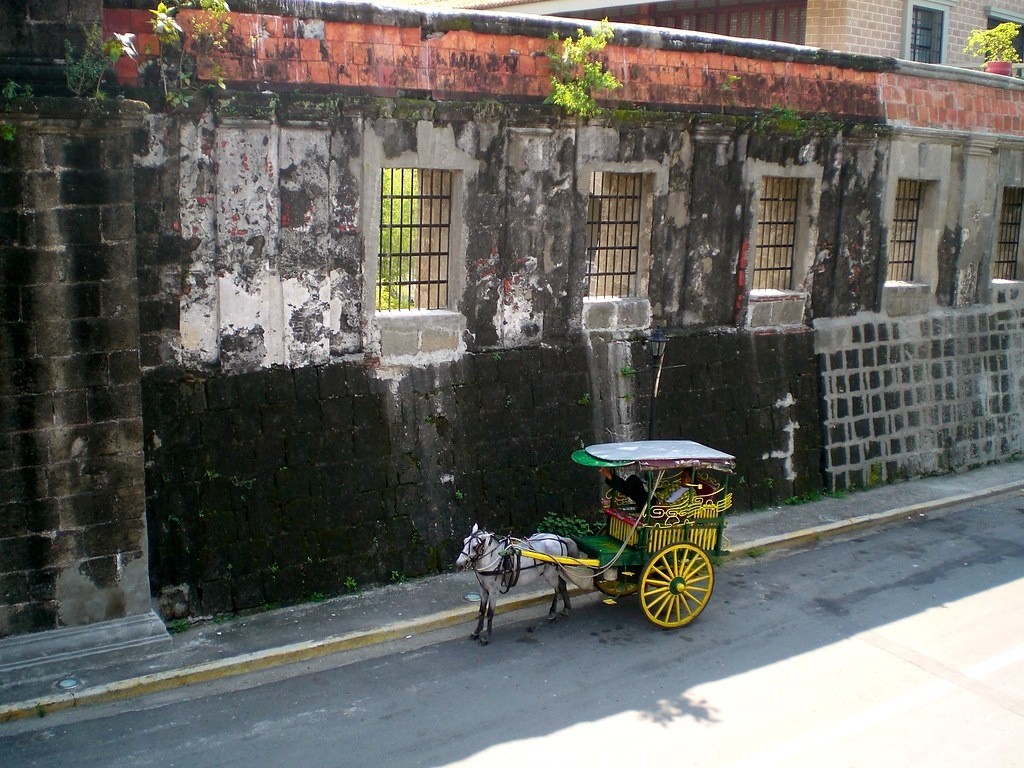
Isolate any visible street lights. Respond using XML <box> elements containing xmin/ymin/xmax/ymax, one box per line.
<box><xmin>644</xmin><ymin>325</ymin><xmax>671</xmax><ymax>440</ymax></box>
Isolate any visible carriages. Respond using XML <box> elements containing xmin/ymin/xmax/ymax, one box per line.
<box><xmin>452</xmin><ymin>438</ymin><xmax>738</xmax><ymax>647</ymax></box>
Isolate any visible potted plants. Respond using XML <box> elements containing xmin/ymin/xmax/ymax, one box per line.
<box><xmin>964</xmin><ymin>20</ymin><xmax>1022</xmax><ymax>76</ymax></box>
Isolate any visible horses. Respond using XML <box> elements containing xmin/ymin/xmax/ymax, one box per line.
<box><xmin>455</xmin><ymin>523</ymin><xmax>580</xmax><ymax>646</ymax></box>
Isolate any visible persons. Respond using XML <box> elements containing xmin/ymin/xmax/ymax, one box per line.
<box><xmin>597</xmin><ymin>466</ymin><xmax>696</xmax><ymax>520</ymax></box>
